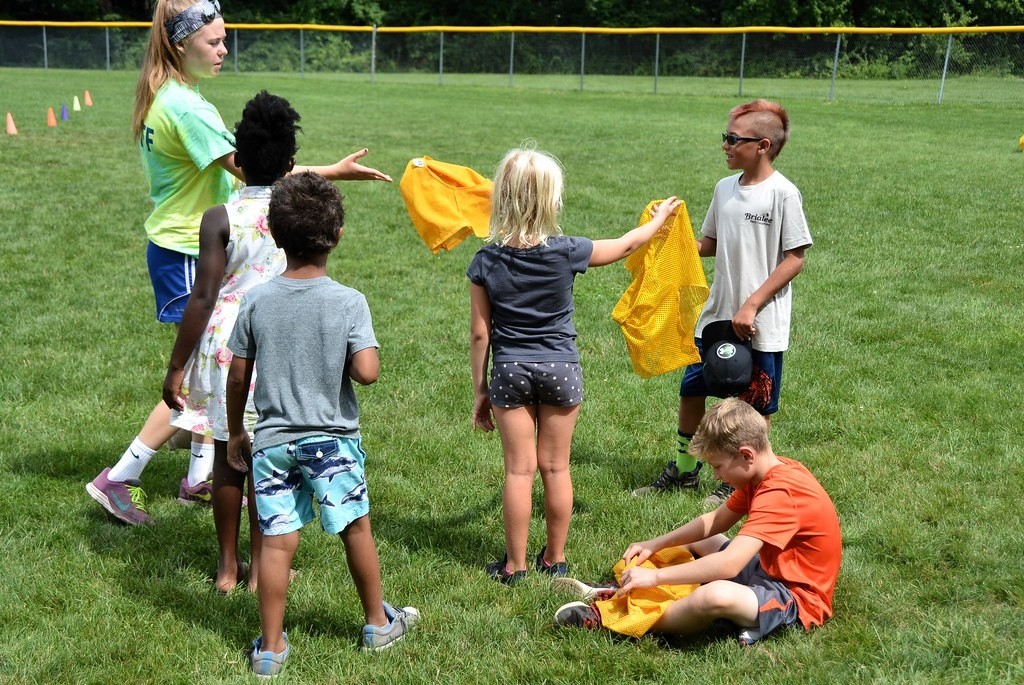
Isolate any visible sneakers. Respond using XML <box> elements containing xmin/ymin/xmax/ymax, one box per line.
<box><xmin>553</xmin><ymin>577</ymin><xmax>620</xmax><ymax>600</ymax></box>
<box><xmin>86</xmin><ymin>467</ymin><xmax>157</xmax><ymax>527</ymax></box>
<box><xmin>554</xmin><ymin>601</ymin><xmax>607</xmax><ymax>630</ymax></box>
<box><xmin>486</xmin><ymin>552</ymin><xmax>527</xmax><ymax>586</ymax></box>
<box><xmin>251</xmin><ymin>631</ymin><xmax>290</xmax><ymax>678</ymax></box>
<box><xmin>709</xmin><ymin>482</ymin><xmax>735</xmax><ymax>502</ymax></box>
<box><xmin>178</xmin><ymin>472</ymin><xmax>249</xmax><ymax>508</ymax></box>
<box><xmin>358</xmin><ymin>601</ymin><xmax>420</xmax><ymax>653</ymax></box>
<box><xmin>535</xmin><ymin>546</ymin><xmax>568</xmax><ymax>575</ymax></box>
<box><xmin>632</xmin><ymin>460</ymin><xmax>702</xmax><ymax>498</ymax></box>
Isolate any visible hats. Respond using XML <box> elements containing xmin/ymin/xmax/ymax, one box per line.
<box><xmin>702</xmin><ymin>320</ymin><xmax>754</xmax><ymax>392</ymax></box>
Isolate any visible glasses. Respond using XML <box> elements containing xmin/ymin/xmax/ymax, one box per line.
<box><xmin>722</xmin><ymin>132</ymin><xmax>773</xmax><ymax>148</ymax></box>
<box><xmin>173</xmin><ymin>0</ymin><xmax>220</xmax><ymax>35</ymax></box>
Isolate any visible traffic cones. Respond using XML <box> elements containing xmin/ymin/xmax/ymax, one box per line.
<box><xmin>5</xmin><ymin>112</ymin><xmax>19</xmax><ymax>135</ymax></box>
<box><xmin>47</xmin><ymin>107</ymin><xmax>60</xmax><ymax>129</ymax></box>
<box><xmin>72</xmin><ymin>96</ymin><xmax>81</xmax><ymax>111</ymax></box>
<box><xmin>83</xmin><ymin>90</ymin><xmax>92</xmax><ymax>106</ymax></box>
<box><xmin>60</xmin><ymin>103</ymin><xmax>70</xmax><ymax>121</ymax></box>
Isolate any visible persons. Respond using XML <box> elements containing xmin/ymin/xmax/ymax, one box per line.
<box><xmin>84</xmin><ymin>0</ymin><xmax>392</xmax><ymax>522</ymax></box>
<box><xmin>632</xmin><ymin>99</ymin><xmax>813</xmax><ymax>506</ymax></box>
<box><xmin>467</xmin><ymin>150</ymin><xmax>684</xmax><ymax>589</ymax></box>
<box><xmin>226</xmin><ymin>172</ymin><xmax>419</xmax><ymax>677</ymax></box>
<box><xmin>163</xmin><ymin>91</ymin><xmax>305</xmax><ymax>594</ymax></box>
<box><xmin>554</xmin><ymin>397</ymin><xmax>843</xmax><ymax>654</ymax></box>
